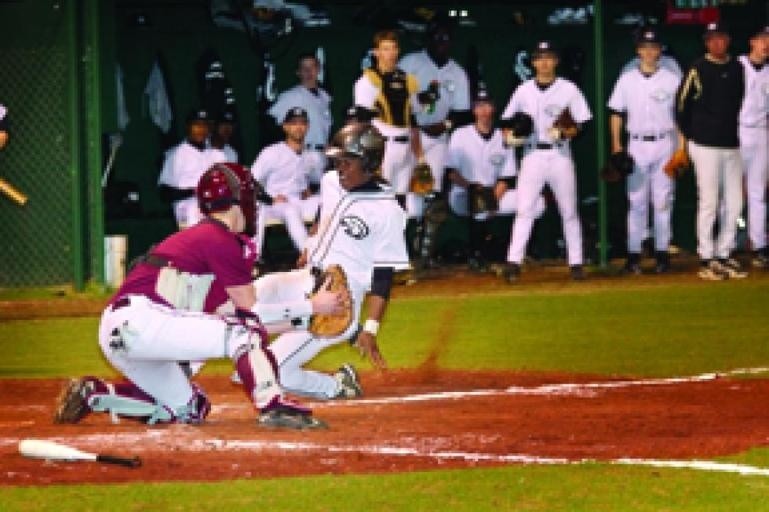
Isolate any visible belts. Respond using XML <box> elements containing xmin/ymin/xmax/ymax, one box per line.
<box><xmin>383</xmin><ymin>135</ymin><xmax>408</xmax><ymax>142</ymax></box>
<box><xmin>630</xmin><ymin>134</ymin><xmax>665</xmax><ymax>142</ymax></box>
<box><xmin>527</xmin><ymin>142</ymin><xmax>563</xmax><ymax>149</ymax></box>
<box><xmin>302</xmin><ymin>142</ymin><xmax>325</xmax><ymax>151</ymax></box>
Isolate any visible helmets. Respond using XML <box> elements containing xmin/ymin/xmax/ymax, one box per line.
<box><xmin>195</xmin><ymin>162</ymin><xmax>274</xmax><ymax>237</ymax></box>
<box><xmin>325</xmin><ymin>120</ymin><xmax>384</xmax><ymax>176</ymax></box>
<box><xmin>184</xmin><ymin>104</ymin><xmax>239</xmax><ymax>141</ymax></box>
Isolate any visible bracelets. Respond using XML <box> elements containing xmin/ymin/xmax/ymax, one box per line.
<box><xmin>363</xmin><ymin>320</ymin><xmax>380</xmax><ymax>337</ymax></box>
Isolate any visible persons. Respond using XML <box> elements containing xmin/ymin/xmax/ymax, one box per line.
<box><xmin>53</xmin><ymin>163</ymin><xmax>347</xmax><ymax>429</ymax></box>
<box><xmin>189</xmin><ymin>120</ymin><xmax>413</xmax><ymax>402</ymax></box>
<box><xmin>0</xmin><ymin>102</ymin><xmax>10</xmax><ymax>151</ymax></box>
<box><xmin>156</xmin><ymin>21</ymin><xmax>768</xmax><ymax>281</ymax></box>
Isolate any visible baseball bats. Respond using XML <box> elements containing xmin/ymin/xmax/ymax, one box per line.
<box><xmin>19</xmin><ymin>439</ymin><xmax>141</xmax><ymax>469</ymax></box>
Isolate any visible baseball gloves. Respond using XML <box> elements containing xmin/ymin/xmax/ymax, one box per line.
<box><xmin>308</xmin><ymin>270</ymin><xmax>351</xmax><ymax>336</ymax></box>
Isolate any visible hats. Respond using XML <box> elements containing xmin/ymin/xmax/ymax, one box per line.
<box><xmin>492</xmin><ymin>112</ymin><xmax>534</xmax><ymax>137</ymax></box>
<box><xmin>528</xmin><ymin>40</ymin><xmax>558</xmax><ymax>58</ymax></box>
<box><xmin>283</xmin><ymin>108</ymin><xmax>308</xmax><ymax>122</ymax></box>
<box><xmin>701</xmin><ymin>21</ymin><xmax>732</xmax><ymax>38</ymax></box>
<box><xmin>471</xmin><ymin>80</ymin><xmax>495</xmax><ymax>101</ymax></box>
<box><xmin>634</xmin><ymin>25</ymin><xmax>666</xmax><ymax>42</ymax></box>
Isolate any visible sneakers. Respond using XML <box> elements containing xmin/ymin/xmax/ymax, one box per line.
<box><xmin>332</xmin><ymin>363</ymin><xmax>363</xmax><ymax>400</ymax></box>
<box><xmin>697</xmin><ymin>253</ymin><xmax>769</xmax><ymax>280</ymax></box>
<box><xmin>256</xmin><ymin>402</ymin><xmax>327</xmax><ymax>430</ymax></box>
<box><xmin>500</xmin><ymin>263</ymin><xmax>521</xmax><ymax>280</ymax></box>
<box><xmin>648</xmin><ymin>261</ymin><xmax>669</xmax><ymax>274</ymax></box>
<box><xmin>570</xmin><ymin>265</ymin><xmax>587</xmax><ymax>281</ymax></box>
<box><xmin>617</xmin><ymin>261</ymin><xmax>642</xmax><ymax>275</ymax></box>
<box><xmin>54</xmin><ymin>376</ymin><xmax>90</xmax><ymax>423</ymax></box>
